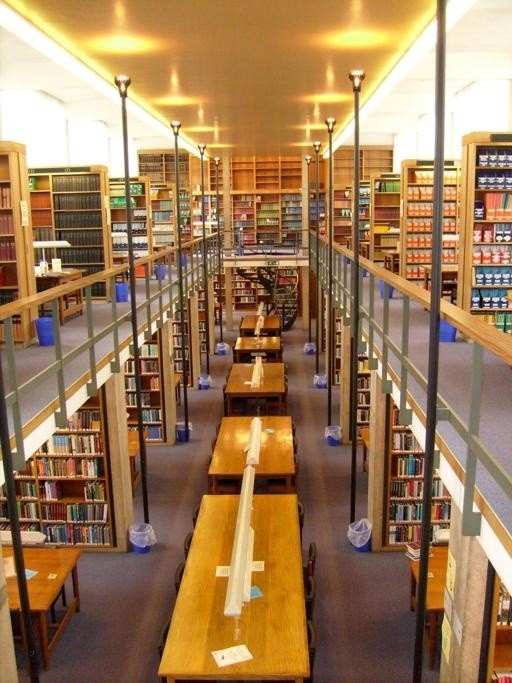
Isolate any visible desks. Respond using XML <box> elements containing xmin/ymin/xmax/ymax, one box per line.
<box><xmin>158</xmin><ymin>494</ymin><xmax>310</xmax><ymax>683</ymax></box>
<box><xmin>2</xmin><ymin>546</ymin><xmax>83</xmax><ymax>671</ymax></box>
<box><xmin>225</xmin><ymin>362</ymin><xmax>285</xmax><ymax>416</ymax></box>
<box><xmin>207</xmin><ymin>416</ymin><xmax>295</xmax><ymax>493</ymax></box>
<box><xmin>35</xmin><ymin>268</ymin><xmax>83</xmax><ymax>326</ymax></box>
<box><xmin>241</xmin><ymin>315</ymin><xmax>280</xmax><ymax>337</ymax></box>
<box><xmin>234</xmin><ymin>336</ymin><xmax>280</xmax><ymax>362</ymax></box>
<box><xmin>358</xmin><ymin>428</ymin><xmax>371</xmax><ymax>472</ymax></box>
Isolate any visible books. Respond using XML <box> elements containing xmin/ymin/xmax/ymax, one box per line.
<box><xmin>232</xmin><ymin>193</ymin><xmax>327</xmax><ymax>320</ymax></box>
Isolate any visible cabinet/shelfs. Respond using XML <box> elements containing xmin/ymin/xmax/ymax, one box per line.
<box><xmin>208</xmin><ymin>154</ymin><xmax>325</xmax><ymax>248</ymax></box>
<box><xmin>1</xmin><ymin>558</ymin><xmax>19</xmax><ymax>683</ymax></box>
<box><xmin>164</xmin><ymin>270</ymin><xmax>222</xmax><ymax>388</ymax></box>
<box><xmin>1</xmin><ymin>319</ymin><xmax>176</xmax><ymax>554</ymax></box>
<box><xmin>1</xmin><ymin>140</ymin><xmax>39</xmax><ymax>350</ymax></box>
<box><xmin>324</xmin><ymin>144</ymin><xmax>404</xmax><ymax>274</ymax></box>
<box><xmin>319</xmin><ymin>291</ymin><xmax>374</xmax><ymax>445</ymax></box>
<box><xmin>366</xmin><ymin>370</ymin><xmax>512</xmax><ymax>683</ymax></box>
<box><xmin>26</xmin><ymin>165</ymin><xmax>177</xmax><ymax>300</ymax></box>
<box><xmin>401</xmin><ymin>133</ymin><xmax>512</xmax><ymax>343</ymax></box>
<box><xmin>139</xmin><ymin>147</ymin><xmax>212</xmax><ymax>256</ymax></box>
<box><xmin>212</xmin><ymin>264</ymin><xmax>302</xmax><ymax>313</ymax></box>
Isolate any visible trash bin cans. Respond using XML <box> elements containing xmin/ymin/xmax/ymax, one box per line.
<box><xmin>343</xmin><ymin>255</ymin><xmax>350</xmax><ymax>264</ymax></box>
<box><xmin>200</xmin><ymin>376</ymin><xmax>209</xmax><ymax>389</ymax></box>
<box><xmin>155</xmin><ymin>266</ymin><xmax>165</xmax><ymax>280</ymax></box>
<box><xmin>176</xmin><ymin>422</ymin><xmax>191</xmax><ymax>441</ymax></box>
<box><xmin>129</xmin><ymin>523</ymin><xmax>152</xmax><ymax>554</ymax></box>
<box><xmin>439</xmin><ymin>320</ymin><xmax>457</xmax><ymax>342</ymax></box>
<box><xmin>362</xmin><ymin>268</ymin><xmax>368</xmax><ymax>277</ymax></box>
<box><xmin>181</xmin><ymin>256</ymin><xmax>187</xmax><ymax>266</ymax></box>
<box><xmin>349</xmin><ymin>520</ymin><xmax>371</xmax><ymax>552</ymax></box>
<box><xmin>380</xmin><ymin>280</ymin><xmax>393</xmax><ymax>298</ymax></box>
<box><xmin>314</xmin><ymin>374</ymin><xmax>327</xmax><ymax>389</ymax></box>
<box><xmin>305</xmin><ymin>343</ymin><xmax>314</xmax><ymax>354</ymax></box>
<box><xmin>217</xmin><ymin>343</ymin><xmax>225</xmax><ymax>355</ymax></box>
<box><xmin>326</xmin><ymin>426</ymin><xmax>341</xmax><ymax>446</ymax></box>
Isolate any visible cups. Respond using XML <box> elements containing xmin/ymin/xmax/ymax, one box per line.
<box><xmin>439</xmin><ymin>317</ymin><xmax>455</xmax><ymax>342</ymax></box>
<box><xmin>155</xmin><ymin>264</ymin><xmax>167</xmax><ymax>280</ymax></box>
<box><xmin>52</xmin><ymin>258</ymin><xmax>62</xmax><ymax>271</ymax></box>
<box><xmin>380</xmin><ymin>279</ymin><xmax>393</xmax><ymax>298</ymax></box>
<box><xmin>343</xmin><ymin>255</ymin><xmax>366</xmax><ymax>277</ymax></box>
<box><xmin>181</xmin><ymin>255</ymin><xmax>187</xmax><ymax>265</ymax></box>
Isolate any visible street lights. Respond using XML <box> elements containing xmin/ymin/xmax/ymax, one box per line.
<box><xmin>198</xmin><ymin>143</ymin><xmax>211</xmax><ymax>381</ymax></box>
<box><xmin>348</xmin><ymin>66</ymin><xmax>366</xmax><ymax>526</ymax></box>
<box><xmin>213</xmin><ymin>156</ymin><xmax>223</xmax><ymax>346</ymax></box>
<box><xmin>311</xmin><ymin>141</ymin><xmax>323</xmax><ymax>373</ymax></box>
<box><xmin>113</xmin><ymin>72</ymin><xmax>150</xmax><ymax>529</ymax></box>
<box><xmin>305</xmin><ymin>153</ymin><xmax>312</xmax><ymax>344</ymax></box>
<box><xmin>325</xmin><ymin>116</ymin><xmax>338</xmax><ymax>427</ymax></box>
<box><xmin>170</xmin><ymin>119</ymin><xmax>190</xmax><ymax>445</ymax></box>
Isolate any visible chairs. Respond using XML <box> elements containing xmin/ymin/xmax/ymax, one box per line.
<box><xmin>156</xmin><ymin>504</ymin><xmax>201</xmax><ymax>683</ymax></box>
<box><xmin>267</xmin><ymin>315</ymin><xmax>283</xmax><ymax>364</ymax></box>
<box><xmin>231</xmin><ymin>316</ymin><xmax>254</xmax><ymax>363</ymax></box>
<box><xmin>264</xmin><ymin>364</ymin><xmax>289</xmax><ymax>416</ymax></box>
<box><xmin>9</xmin><ymin>608</ymin><xmax>48</xmax><ymax>678</ymax></box>
<box><xmin>206</xmin><ymin>423</ymin><xmax>239</xmax><ymax>494</ymax></box>
<box><xmin>267</xmin><ymin>421</ymin><xmax>299</xmax><ymax>493</ymax></box>
<box><xmin>296</xmin><ymin>502</ymin><xmax>316</xmax><ymax>683</ymax></box>
<box><xmin>222</xmin><ymin>362</ymin><xmax>247</xmax><ymax>415</ymax></box>
<box><xmin>50</xmin><ymin>581</ymin><xmax>67</xmax><ymax>624</ymax></box>
